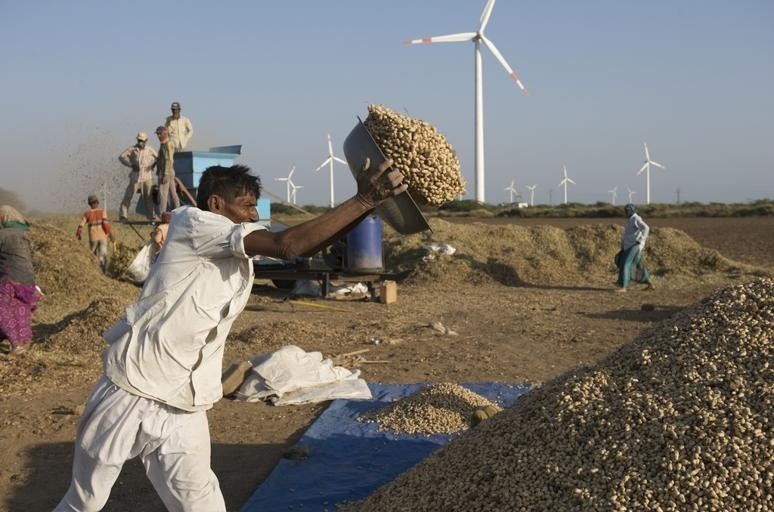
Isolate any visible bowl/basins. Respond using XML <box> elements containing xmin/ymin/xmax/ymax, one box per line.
<box><xmin>342</xmin><ymin>114</ymin><xmax>435</xmax><ymax>236</ymax></box>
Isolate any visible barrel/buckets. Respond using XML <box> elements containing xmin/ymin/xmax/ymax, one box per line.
<box><xmin>345</xmin><ymin>215</ymin><xmax>383</xmax><ymax>269</ymax></box>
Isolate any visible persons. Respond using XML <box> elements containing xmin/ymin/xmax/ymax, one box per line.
<box><xmin>162</xmin><ymin>101</ymin><xmax>193</xmax><ymax>153</ymax></box>
<box><xmin>145</xmin><ymin>126</ymin><xmax>180</xmax><ymax>222</ymax></box>
<box><xmin>148</xmin><ymin>211</ymin><xmax>169</xmax><ymax>249</ymax></box>
<box><xmin>117</xmin><ymin>132</ymin><xmax>158</xmax><ymax>221</ymax></box>
<box><xmin>0</xmin><ymin>205</ymin><xmax>43</xmax><ymax>356</ymax></box>
<box><xmin>611</xmin><ymin>203</ymin><xmax>655</xmax><ymax>293</ymax></box>
<box><xmin>75</xmin><ymin>194</ymin><xmax>115</xmax><ymax>273</ymax></box>
<box><xmin>43</xmin><ymin>155</ymin><xmax>412</xmax><ymax>511</ymax></box>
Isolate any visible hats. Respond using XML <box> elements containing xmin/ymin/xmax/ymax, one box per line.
<box><xmin>136</xmin><ymin>132</ymin><xmax>149</xmax><ymax>140</ymax></box>
<box><xmin>88</xmin><ymin>195</ymin><xmax>99</xmax><ymax>203</ymax></box>
<box><xmin>172</xmin><ymin>102</ymin><xmax>181</xmax><ymax>110</ymax></box>
<box><xmin>155</xmin><ymin>126</ymin><xmax>167</xmax><ymax>133</ymax></box>
